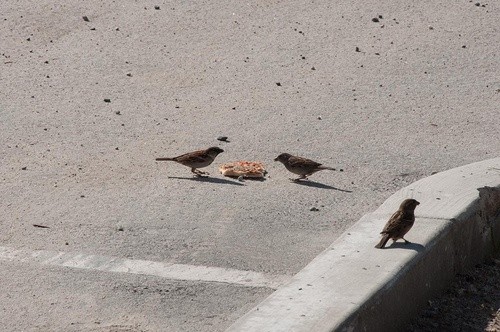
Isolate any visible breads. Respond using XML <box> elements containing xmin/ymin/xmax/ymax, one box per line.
<box><xmin>221</xmin><ymin>161</ymin><xmax>266</xmax><ymax>178</ymax></box>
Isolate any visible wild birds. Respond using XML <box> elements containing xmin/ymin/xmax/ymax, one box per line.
<box><xmin>374</xmin><ymin>199</ymin><xmax>420</xmax><ymax>249</ymax></box>
<box><xmin>156</xmin><ymin>147</ymin><xmax>224</xmax><ymax>178</ymax></box>
<box><xmin>275</xmin><ymin>153</ymin><xmax>336</xmax><ymax>181</ymax></box>
